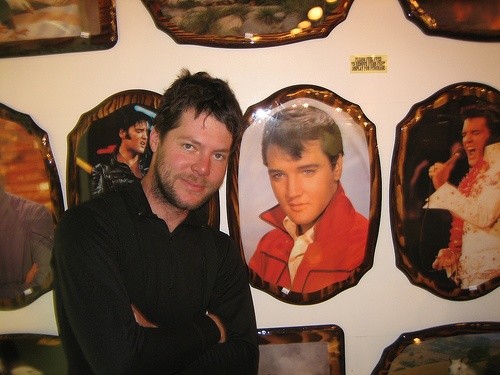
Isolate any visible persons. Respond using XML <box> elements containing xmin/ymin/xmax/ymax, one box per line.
<box><xmin>422</xmin><ymin>103</ymin><xmax>500</xmax><ymax>291</ymax></box>
<box><xmin>51</xmin><ymin>68</ymin><xmax>260</xmax><ymax>375</ymax></box>
<box><xmin>249</xmin><ymin>105</ymin><xmax>371</xmax><ymax>294</ymax></box>
<box><xmin>0</xmin><ymin>182</ymin><xmax>56</xmax><ymax>308</ymax></box>
<box><xmin>90</xmin><ymin>111</ymin><xmax>149</xmax><ymax>199</ymax></box>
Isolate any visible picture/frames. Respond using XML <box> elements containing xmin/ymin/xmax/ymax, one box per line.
<box><xmin>66</xmin><ymin>89</ymin><xmax>220</xmax><ymax>232</ymax></box>
<box><xmin>226</xmin><ymin>84</ymin><xmax>382</xmax><ymax>306</ymax></box>
<box><xmin>141</xmin><ymin>0</ymin><xmax>354</xmax><ymax>49</ymax></box>
<box><xmin>0</xmin><ymin>103</ymin><xmax>65</xmax><ymax>312</ymax></box>
<box><xmin>369</xmin><ymin>321</ymin><xmax>500</xmax><ymax>375</ymax></box>
<box><xmin>398</xmin><ymin>0</ymin><xmax>500</xmax><ymax>43</ymax></box>
<box><xmin>0</xmin><ymin>0</ymin><xmax>118</xmax><ymax>58</ymax></box>
<box><xmin>388</xmin><ymin>82</ymin><xmax>500</xmax><ymax>301</ymax></box>
<box><xmin>258</xmin><ymin>324</ymin><xmax>346</xmax><ymax>375</ymax></box>
<box><xmin>0</xmin><ymin>333</ymin><xmax>72</xmax><ymax>375</ymax></box>
